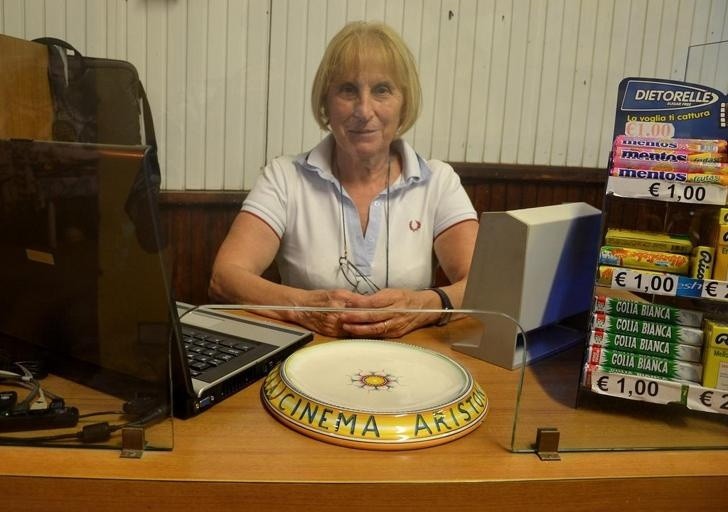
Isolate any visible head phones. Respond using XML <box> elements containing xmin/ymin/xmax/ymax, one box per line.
<box><xmin>35</xmin><ymin>34</ymin><xmax>87</xmax><ymax>140</ymax></box>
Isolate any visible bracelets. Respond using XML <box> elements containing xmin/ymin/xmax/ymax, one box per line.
<box><xmin>425</xmin><ymin>283</ymin><xmax>455</xmax><ymax>328</ymax></box>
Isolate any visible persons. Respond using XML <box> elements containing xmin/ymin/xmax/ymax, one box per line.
<box><xmin>208</xmin><ymin>21</ymin><xmax>480</xmax><ymax>343</ymax></box>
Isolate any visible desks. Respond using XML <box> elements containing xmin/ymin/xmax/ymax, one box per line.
<box><xmin>0</xmin><ymin>307</ymin><xmax>728</xmax><ymax>511</ymax></box>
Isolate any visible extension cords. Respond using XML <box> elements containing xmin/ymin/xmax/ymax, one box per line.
<box><xmin>0</xmin><ymin>406</ymin><xmax>81</xmax><ymax>431</ymax></box>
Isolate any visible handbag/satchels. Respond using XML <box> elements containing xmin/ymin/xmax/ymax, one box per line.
<box><xmin>65</xmin><ymin>53</ymin><xmax>161</xmax><ymax>196</ymax></box>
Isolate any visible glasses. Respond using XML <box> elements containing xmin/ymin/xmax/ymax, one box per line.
<box><xmin>340</xmin><ymin>255</ymin><xmax>381</xmax><ymax>298</ymax></box>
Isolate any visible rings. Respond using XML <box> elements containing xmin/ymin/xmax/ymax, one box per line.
<box><xmin>384</xmin><ymin>319</ymin><xmax>390</xmax><ymax>334</ymax></box>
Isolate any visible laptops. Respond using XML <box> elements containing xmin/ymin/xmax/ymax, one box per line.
<box><xmin>0</xmin><ymin>138</ymin><xmax>314</xmax><ymax>422</ymax></box>
